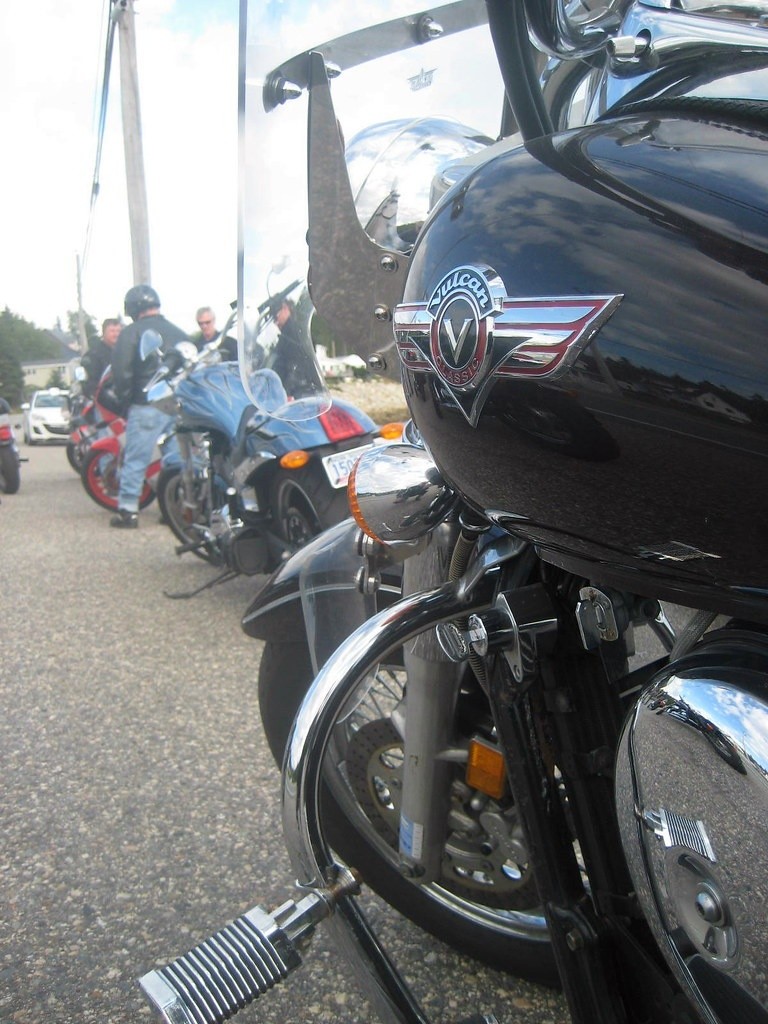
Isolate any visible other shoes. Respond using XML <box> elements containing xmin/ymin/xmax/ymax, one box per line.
<box><xmin>159</xmin><ymin>517</ymin><xmax>169</xmax><ymax>525</ymax></box>
<box><xmin>109</xmin><ymin>513</ymin><xmax>139</xmax><ymax>529</ymax></box>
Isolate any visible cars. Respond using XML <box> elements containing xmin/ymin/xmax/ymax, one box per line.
<box><xmin>19</xmin><ymin>387</ymin><xmax>81</xmax><ymax>446</ymax></box>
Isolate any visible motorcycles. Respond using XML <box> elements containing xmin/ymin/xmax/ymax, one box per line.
<box><xmin>138</xmin><ymin>276</ymin><xmax>407</xmax><ymax>601</ymax></box>
<box><xmin>0</xmin><ymin>383</ymin><xmax>31</xmax><ymax>494</ymax></box>
<box><xmin>66</xmin><ymin>364</ymin><xmax>173</xmax><ymax>512</ymax></box>
<box><xmin>139</xmin><ymin>2</ymin><xmax>768</xmax><ymax>1023</ymax></box>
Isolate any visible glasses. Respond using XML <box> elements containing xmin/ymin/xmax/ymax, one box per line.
<box><xmin>272</xmin><ymin>311</ymin><xmax>281</xmax><ymax>324</ymax></box>
<box><xmin>198</xmin><ymin>320</ymin><xmax>212</xmax><ymax>326</ymax></box>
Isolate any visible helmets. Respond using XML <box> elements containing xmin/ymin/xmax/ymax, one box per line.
<box><xmin>124</xmin><ymin>284</ymin><xmax>161</xmax><ymax>322</ymax></box>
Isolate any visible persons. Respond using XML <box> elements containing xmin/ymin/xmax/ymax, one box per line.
<box><xmin>193</xmin><ymin>306</ymin><xmax>239</xmax><ymax>364</ymax></box>
<box><xmin>106</xmin><ymin>283</ymin><xmax>197</xmax><ymax>529</ymax></box>
<box><xmin>266</xmin><ymin>294</ymin><xmax>316</xmax><ymax>404</ymax></box>
<box><xmin>78</xmin><ymin>316</ymin><xmax>126</xmax><ymax>397</ymax></box>
